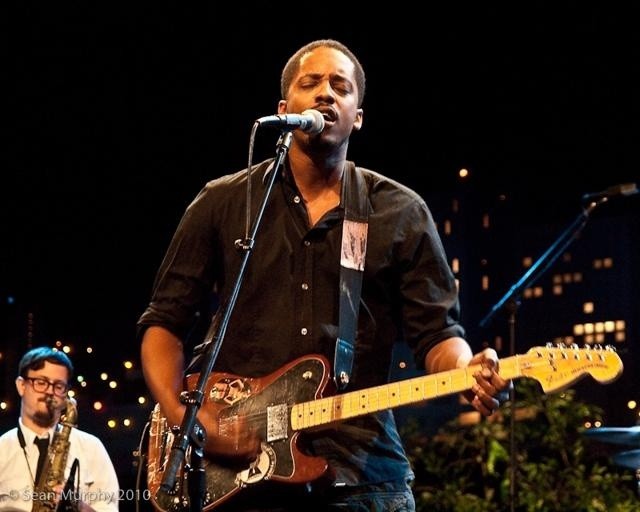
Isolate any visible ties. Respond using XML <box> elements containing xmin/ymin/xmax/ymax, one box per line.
<box><xmin>34</xmin><ymin>432</ymin><xmax>50</xmax><ymax>486</ymax></box>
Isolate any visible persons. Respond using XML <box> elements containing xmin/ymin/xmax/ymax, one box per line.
<box><xmin>0</xmin><ymin>347</ymin><xmax>120</xmax><ymax>512</ymax></box>
<box><xmin>136</xmin><ymin>39</ymin><xmax>513</xmax><ymax>512</ymax></box>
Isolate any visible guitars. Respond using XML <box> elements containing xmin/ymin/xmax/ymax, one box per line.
<box><xmin>147</xmin><ymin>340</ymin><xmax>625</xmax><ymax>512</ymax></box>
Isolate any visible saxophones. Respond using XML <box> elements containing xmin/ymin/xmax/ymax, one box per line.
<box><xmin>30</xmin><ymin>397</ymin><xmax>79</xmax><ymax>512</ymax></box>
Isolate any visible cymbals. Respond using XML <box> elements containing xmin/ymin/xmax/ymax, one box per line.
<box><xmin>582</xmin><ymin>424</ymin><xmax>640</xmax><ymax>446</ymax></box>
<box><xmin>613</xmin><ymin>449</ymin><xmax>640</xmax><ymax>471</ymax></box>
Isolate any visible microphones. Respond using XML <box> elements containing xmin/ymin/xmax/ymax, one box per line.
<box><xmin>257</xmin><ymin>109</ymin><xmax>324</xmax><ymax>135</ymax></box>
<box><xmin>583</xmin><ymin>183</ymin><xmax>637</xmax><ymax>200</ymax></box>
<box><xmin>56</xmin><ymin>459</ymin><xmax>78</xmax><ymax>512</ymax></box>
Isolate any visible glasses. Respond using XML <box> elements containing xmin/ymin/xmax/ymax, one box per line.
<box><xmin>26</xmin><ymin>377</ymin><xmax>72</xmax><ymax>397</ymax></box>
